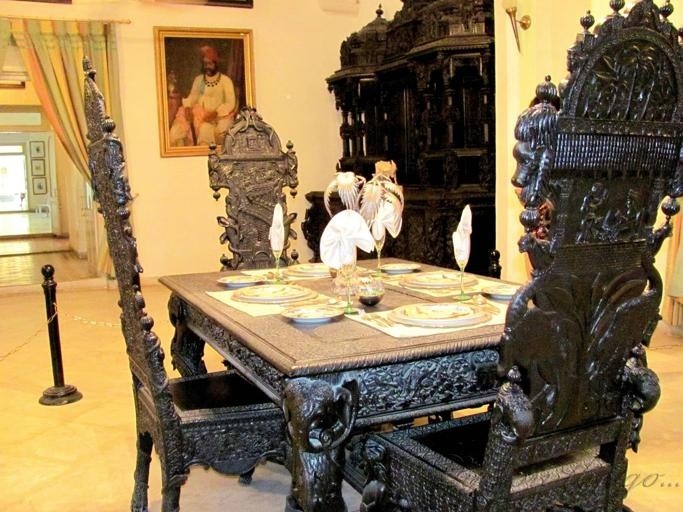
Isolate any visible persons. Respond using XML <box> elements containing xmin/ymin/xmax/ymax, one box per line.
<box><xmin>180</xmin><ymin>43</ymin><xmax>236</xmax><ymax>145</ymax></box>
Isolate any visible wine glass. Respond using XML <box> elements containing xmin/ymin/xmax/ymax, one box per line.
<box><xmin>371</xmin><ymin>224</ymin><xmax>385</xmax><ymax>272</ymax></box>
<box><xmin>336</xmin><ymin>246</ymin><xmax>358</xmax><ymax>314</ymax></box>
<box><xmin>451</xmin><ymin>231</ymin><xmax>472</xmax><ymax>300</ymax></box>
<box><xmin>269</xmin><ymin>227</ymin><xmax>284</xmax><ymax>276</ymax></box>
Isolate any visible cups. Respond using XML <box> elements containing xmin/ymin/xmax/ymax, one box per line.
<box><xmin>358</xmin><ymin>277</ymin><xmax>384</xmax><ymax>307</ymax></box>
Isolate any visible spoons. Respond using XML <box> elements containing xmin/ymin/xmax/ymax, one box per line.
<box><xmin>361</xmin><ymin>312</ymin><xmax>390</xmax><ymax>328</ymax></box>
<box><xmin>473</xmin><ymin>294</ymin><xmax>497</xmax><ymax>311</ymax></box>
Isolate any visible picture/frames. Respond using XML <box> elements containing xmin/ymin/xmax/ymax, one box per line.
<box><xmin>29</xmin><ymin>141</ymin><xmax>47</xmax><ymax>195</ymax></box>
<box><xmin>153</xmin><ymin>0</ymin><xmax>253</xmax><ymax>8</ymax></box>
<box><xmin>152</xmin><ymin>25</ymin><xmax>256</xmax><ymax>160</ymax></box>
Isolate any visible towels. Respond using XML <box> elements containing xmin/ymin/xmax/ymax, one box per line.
<box><xmin>316</xmin><ymin>209</ymin><xmax>373</xmax><ymax>267</ymax></box>
<box><xmin>450</xmin><ymin>203</ymin><xmax>473</xmax><ymax>263</ymax></box>
<box><xmin>265</xmin><ymin>203</ymin><xmax>284</xmax><ymax>252</ymax></box>
<box><xmin>372</xmin><ymin>199</ymin><xmax>404</xmax><ymax>241</ymax></box>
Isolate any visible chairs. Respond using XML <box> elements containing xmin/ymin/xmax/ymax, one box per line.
<box><xmin>72</xmin><ymin>50</ymin><xmax>292</xmax><ymax>512</ymax></box>
<box><xmin>203</xmin><ymin>102</ymin><xmax>300</xmax><ymax>489</ymax></box>
<box><xmin>338</xmin><ymin>1</ymin><xmax>681</xmax><ymax>512</ymax></box>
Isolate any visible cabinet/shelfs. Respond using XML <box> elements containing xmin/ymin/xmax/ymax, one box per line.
<box><xmin>302</xmin><ymin>0</ymin><xmax>500</xmax><ymax>285</ymax></box>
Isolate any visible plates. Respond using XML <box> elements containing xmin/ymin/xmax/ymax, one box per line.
<box><xmin>389</xmin><ymin>303</ymin><xmax>490</xmax><ymax>329</ymax></box>
<box><xmin>481</xmin><ymin>285</ymin><xmax>516</xmax><ymax>300</ymax></box>
<box><xmin>378</xmin><ymin>263</ymin><xmax>419</xmax><ymax>274</ymax></box>
<box><xmin>216</xmin><ymin>276</ymin><xmax>264</xmax><ymax>288</ymax></box>
<box><xmin>281</xmin><ymin>303</ymin><xmax>346</xmax><ymax>324</ymax></box>
<box><xmin>397</xmin><ymin>272</ymin><xmax>476</xmax><ymax>289</ymax></box>
<box><xmin>231</xmin><ymin>284</ymin><xmax>317</xmax><ymax>304</ymax></box>
<box><xmin>282</xmin><ymin>263</ymin><xmax>329</xmax><ymax>277</ymax></box>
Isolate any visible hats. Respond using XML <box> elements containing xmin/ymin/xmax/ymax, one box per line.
<box><xmin>200</xmin><ymin>45</ymin><xmax>220</xmax><ymax>65</ymax></box>
